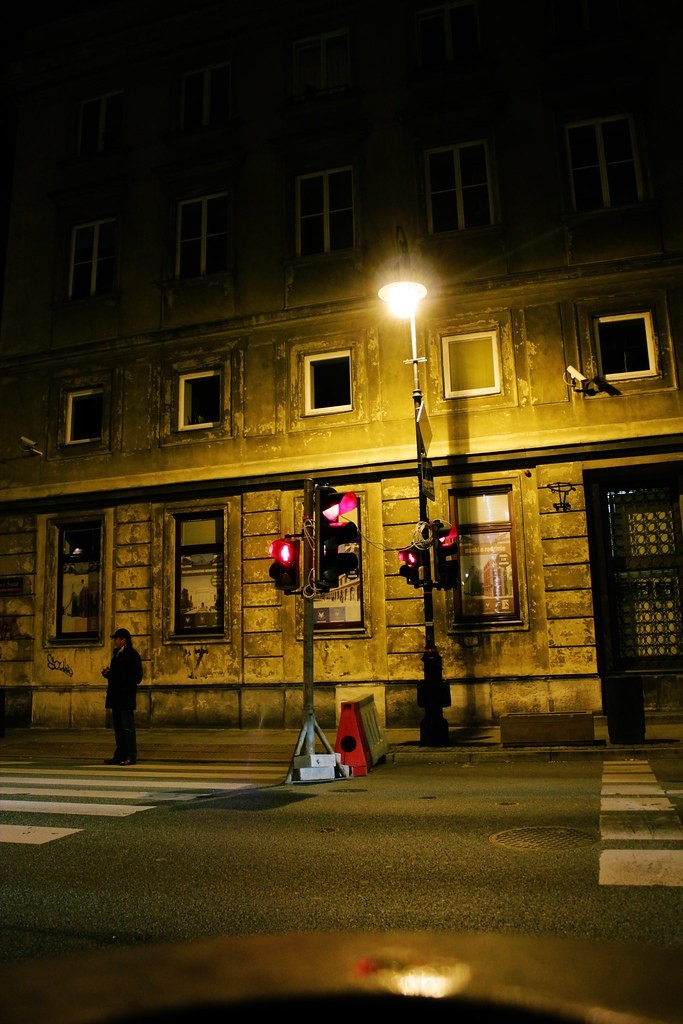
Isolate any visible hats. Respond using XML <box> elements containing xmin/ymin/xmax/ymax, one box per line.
<box><xmin>110</xmin><ymin>628</ymin><xmax>131</xmax><ymax>638</ymax></box>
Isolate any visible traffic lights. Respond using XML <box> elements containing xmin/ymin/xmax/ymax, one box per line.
<box><xmin>430</xmin><ymin>522</ymin><xmax>456</xmax><ymax>587</ymax></box>
<box><xmin>398</xmin><ymin>544</ymin><xmax>424</xmax><ymax>586</ymax></box>
<box><xmin>269</xmin><ymin>537</ymin><xmax>297</xmax><ymax>588</ymax></box>
<box><xmin>314</xmin><ymin>483</ymin><xmax>360</xmax><ymax>589</ymax></box>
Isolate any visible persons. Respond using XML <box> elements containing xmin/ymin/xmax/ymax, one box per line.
<box><xmin>100</xmin><ymin>628</ymin><xmax>143</xmax><ymax>765</ymax></box>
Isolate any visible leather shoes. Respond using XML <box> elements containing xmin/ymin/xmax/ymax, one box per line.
<box><xmin>104</xmin><ymin>756</ymin><xmax>120</xmax><ymax>764</ymax></box>
<box><xmin>120</xmin><ymin>759</ymin><xmax>135</xmax><ymax>765</ymax></box>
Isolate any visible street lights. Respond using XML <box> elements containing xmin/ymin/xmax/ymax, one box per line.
<box><xmin>379</xmin><ymin>224</ymin><xmax>453</xmax><ymax>747</ymax></box>
